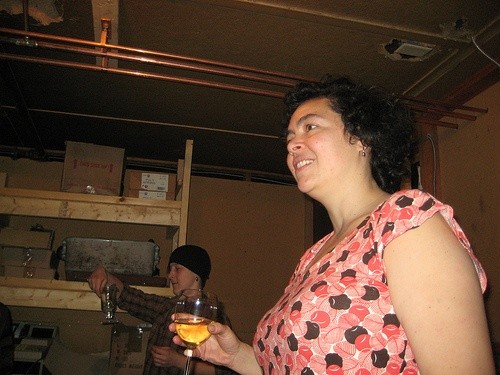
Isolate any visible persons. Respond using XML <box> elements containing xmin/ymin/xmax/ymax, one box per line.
<box><xmin>89</xmin><ymin>245</ymin><xmax>234</xmax><ymax>375</ymax></box>
<box><xmin>169</xmin><ymin>71</ymin><xmax>497</xmax><ymax>375</ymax></box>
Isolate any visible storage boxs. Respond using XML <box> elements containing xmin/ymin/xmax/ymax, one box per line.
<box><xmin>0</xmin><ymin>229</ymin><xmax>56</xmax><ymax>279</ymax></box>
<box><xmin>14</xmin><ymin>338</ymin><xmax>49</xmax><ymax>360</ymax></box>
<box><xmin>122</xmin><ymin>169</ymin><xmax>177</xmax><ymax>200</ymax></box>
<box><xmin>31</xmin><ymin>163</ymin><xmax>63</xmax><ymax>191</ymax></box>
<box><xmin>62</xmin><ymin>141</ymin><xmax>125</xmax><ymax>195</ymax></box>
<box><xmin>108</xmin><ymin>326</ymin><xmax>151</xmax><ymax>375</ymax></box>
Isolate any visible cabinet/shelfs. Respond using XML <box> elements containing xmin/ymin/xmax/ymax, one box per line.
<box><xmin>0</xmin><ymin>139</ymin><xmax>193</xmax><ymax>311</ymax></box>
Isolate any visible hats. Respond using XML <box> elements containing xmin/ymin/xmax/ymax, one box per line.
<box><xmin>168</xmin><ymin>245</ymin><xmax>212</xmax><ymax>290</ymax></box>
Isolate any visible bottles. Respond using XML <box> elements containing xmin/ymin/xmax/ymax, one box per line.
<box><xmin>24</xmin><ymin>246</ymin><xmax>33</xmax><ymax>279</ymax></box>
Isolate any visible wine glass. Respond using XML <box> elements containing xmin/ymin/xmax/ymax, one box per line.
<box><xmin>174</xmin><ymin>289</ymin><xmax>219</xmax><ymax>375</ymax></box>
<box><xmin>100</xmin><ymin>285</ymin><xmax>120</xmax><ymax>324</ymax></box>
